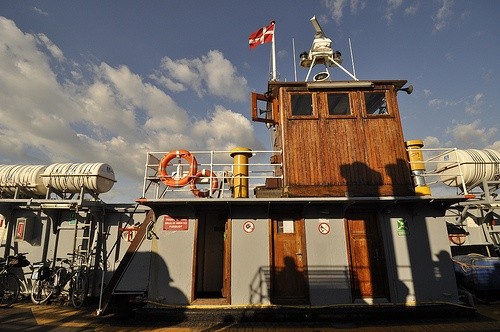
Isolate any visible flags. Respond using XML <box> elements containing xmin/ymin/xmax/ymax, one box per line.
<box><xmin>248</xmin><ymin>20</ymin><xmax>275</xmax><ymax>49</ymax></box>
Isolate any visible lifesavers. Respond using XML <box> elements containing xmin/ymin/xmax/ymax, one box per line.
<box><xmin>190</xmin><ymin>170</ymin><xmax>218</xmax><ymax>197</ymax></box>
<box><xmin>158</xmin><ymin>150</ymin><xmax>197</xmax><ymax>187</ymax></box>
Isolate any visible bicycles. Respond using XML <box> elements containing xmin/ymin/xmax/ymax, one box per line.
<box><xmin>31</xmin><ymin>253</ymin><xmax>96</xmax><ymax>309</ymax></box>
<box><xmin>0</xmin><ymin>254</ymin><xmax>21</xmax><ymax>309</ymax></box>
